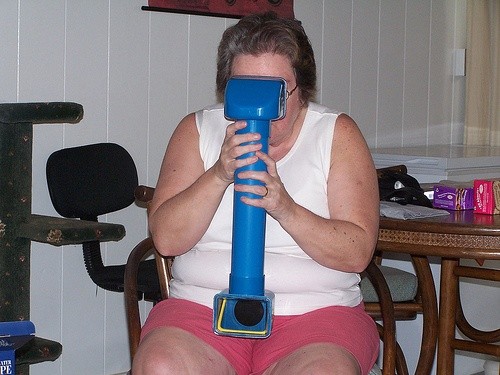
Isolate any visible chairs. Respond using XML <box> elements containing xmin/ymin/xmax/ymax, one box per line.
<box><xmin>45</xmin><ymin>142</ymin><xmax>176</xmax><ymax>309</ymax></box>
<box><xmin>123</xmin><ymin>185</ymin><xmax>439</xmax><ymax>375</ymax></box>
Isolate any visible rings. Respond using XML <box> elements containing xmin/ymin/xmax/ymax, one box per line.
<box><xmin>261</xmin><ymin>185</ymin><xmax>269</xmax><ymax>198</ymax></box>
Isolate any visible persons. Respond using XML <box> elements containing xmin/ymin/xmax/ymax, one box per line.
<box><xmin>130</xmin><ymin>12</ymin><xmax>380</xmax><ymax>375</ymax></box>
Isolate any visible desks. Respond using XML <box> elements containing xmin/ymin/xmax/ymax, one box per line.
<box><xmin>376</xmin><ymin>207</ymin><xmax>500</xmax><ymax>375</ymax></box>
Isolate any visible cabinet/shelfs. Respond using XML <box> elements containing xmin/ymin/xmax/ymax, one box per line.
<box><xmin>0</xmin><ymin>102</ymin><xmax>99</xmax><ymax>375</ymax></box>
